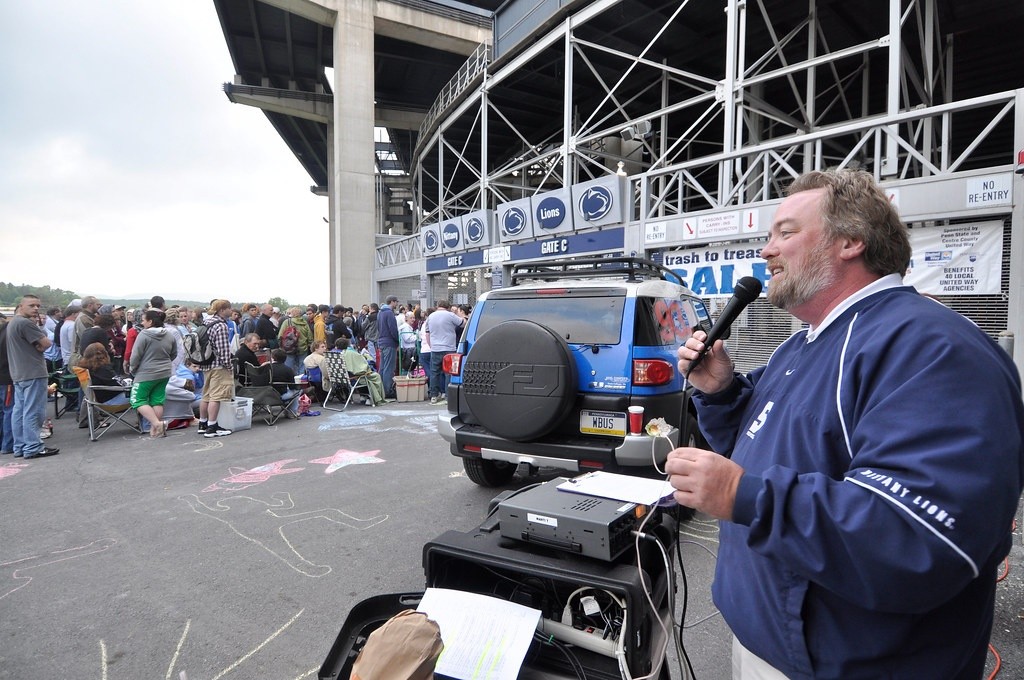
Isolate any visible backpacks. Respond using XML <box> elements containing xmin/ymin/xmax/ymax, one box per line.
<box><xmin>281</xmin><ymin>318</ymin><xmax>300</xmax><ymax>354</ymax></box>
<box><xmin>184</xmin><ymin>319</ymin><xmax>228</xmax><ymax>366</ymax></box>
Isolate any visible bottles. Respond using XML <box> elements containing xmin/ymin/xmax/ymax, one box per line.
<box><xmin>294</xmin><ymin>376</ymin><xmax>309</xmax><ymax>384</ymax></box>
<box><xmin>46</xmin><ymin>418</ymin><xmax>53</xmax><ymax>434</ymax></box>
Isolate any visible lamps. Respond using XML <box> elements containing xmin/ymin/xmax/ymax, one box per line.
<box><xmin>619</xmin><ymin>119</ymin><xmax>650</xmax><ymax>141</ymax></box>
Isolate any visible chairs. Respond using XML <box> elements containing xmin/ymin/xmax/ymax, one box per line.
<box><xmin>49</xmin><ymin>358</ymin><xmax>80</xmax><ymax>419</ymax></box>
<box><xmin>230</xmin><ymin>358</ymin><xmax>270</xmax><ymax>413</ymax></box>
<box><xmin>321</xmin><ymin>350</ymin><xmax>376</xmax><ymax>412</ymax></box>
<box><xmin>302</xmin><ymin>365</ymin><xmax>344</xmax><ymax>405</ymax></box>
<box><xmin>243</xmin><ymin>361</ymin><xmax>300</xmax><ymax>425</ymax></box>
<box><xmin>72</xmin><ymin>366</ymin><xmax>143</xmax><ymax>441</ymax></box>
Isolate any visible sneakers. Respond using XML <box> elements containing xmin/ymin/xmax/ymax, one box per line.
<box><xmin>204</xmin><ymin>421</ymin><xmax>232</xmax><ymax>437</ymax></box>
<box><xmin>197</xmin><ymin>420</ymin><xmax>208</xmax><ymax>433</ymax></box>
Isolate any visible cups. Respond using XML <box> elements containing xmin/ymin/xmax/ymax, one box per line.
<box><xmin>123</xmin><ymin>379</ymin><xmax>132</xmax><ymax>386</ymax></box>
<box><xmin>628</xmin><ymin>406</ymin><xmax>645</xmax><ymax>435</ymax></box>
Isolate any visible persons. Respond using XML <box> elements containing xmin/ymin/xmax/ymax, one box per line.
<box><xmin>665</xmin><ymin>166</ymin><xmax>1024</xmax><ymax>680</ymax></box>
<box><xmin>0</xmin><ymin>294</ymin><xmax>473</xmax><ymax>460</ymax></box>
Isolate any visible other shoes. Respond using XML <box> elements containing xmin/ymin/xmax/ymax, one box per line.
<box><xmin>384</xmin><ymin>394</ymin><xmax>397</xmax><ymax>401</ymax></box>
<box><xmin>431</xmin><ymin>392</ymin><xmax>445</xmax><ymax>403</ymax></box>
<box><xmin>25</xmin><ymin>447</ymin><xmax>60</xmax><ymax>459</ymax></box>
<box><xmin>40</xmin><ymin>431</ymin><xmax>52</xmax><ymax>438</ymax></box>
<box><xmin>50</xmin><ymin>391</ymin><xmax>63</xmax><ymax>398</ymax></box>
<box><xmin>94</xmin><ymin>421</ymin><xmax>111</xmax><ymax>428</ymax></box>
<box><xmin>286</xmin><ymin>412</ymin><xmax>301</xmax><ymax>418</ymax></box>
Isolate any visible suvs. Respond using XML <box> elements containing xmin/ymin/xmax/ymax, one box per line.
<box><xmin>437</xmin><ymin>258</ymin><xmax>733</xmax><ymax>489</ymax></box>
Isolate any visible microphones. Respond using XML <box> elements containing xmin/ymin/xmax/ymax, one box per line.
<box><xmin>688</xmin><ymin>276</ymin><xmax>762</xmax><ymax>370</ymax></box>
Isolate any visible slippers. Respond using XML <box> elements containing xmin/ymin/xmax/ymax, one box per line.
<box><xmin>151</xmin><ymin>420</ymin><xmax>169</xmax><ymax>438</ymax></box>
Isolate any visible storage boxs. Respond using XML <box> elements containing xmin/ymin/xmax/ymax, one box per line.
<box><xmin>393</xmin><ymin>375</ymin><xmax>425</xmax><ymax>402</ymax></box>
<box><xmin>217</xmin><ymin>395</ymin><xmax>254</xmax><ymax>431</ymax></box>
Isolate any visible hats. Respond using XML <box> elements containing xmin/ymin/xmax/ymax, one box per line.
<box><xmin>272</xmin><ymin>307</ymin><xmax>280</xmax><ymax>313</ymax></box>
<box><xmin>386</xmin><ymin>295</ymin><xmax>398</xmax><ymax>301</ymax></box>
<box><xmin>115</xmin><ymin>305</ymin><xmax>126</xmax><ymax>310</ymax></box>
<box><xmin>207</xmin><ymin>300</ymin><xmax>230</xmax><ymax>315</ymax></box>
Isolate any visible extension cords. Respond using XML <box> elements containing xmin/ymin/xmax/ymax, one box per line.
<box><xmin>538</xmin><ymin>613</ymin><xmax>621</xmax><ymax>659</ymax></box>
